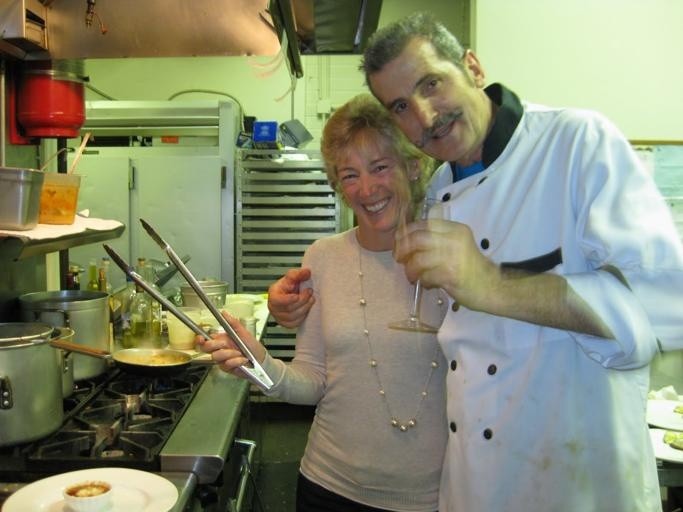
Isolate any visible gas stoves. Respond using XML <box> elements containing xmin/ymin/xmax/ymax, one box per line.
<box><xmin>0</xmin><ymin>366</ymin><xmax>246</xmax><ymax>483</ymax></box>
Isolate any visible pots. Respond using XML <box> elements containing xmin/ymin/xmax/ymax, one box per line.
<box><xmin>108</xmin><ymin>348</ymin><xmax>212</xmax><ymax>372</ymax></box>
<box><xmin>0</xmin><ymin>325</ymin><xmax>64</xmax><ymax>447</ymax></box>
<box><xmin>59</xmin><ymin>327</ymin><xmax>77</xmax><ymax>400</ymax></box>
<box><xmin>17</xmin><ymin>287</ymin><xmax>109</xmax><ymax>382</ymax></box>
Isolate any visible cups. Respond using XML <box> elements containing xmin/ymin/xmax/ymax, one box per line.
<box><xmin>42</xmin><ymin>170</ymin><xmax>82</xmax><ymax>226</ymax></box>
<box><xmin>63</xmin><ymin>479</ymin><xmax>112</xmax><ymax>512</ymax></box>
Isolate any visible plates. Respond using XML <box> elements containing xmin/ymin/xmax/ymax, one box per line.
<box><xmin>0</xmin><ymin>465</ymin><xmax>180</xmax><ymax>512</ymax></box>
<box><xmin>645</xmin><ymin>398</ymin><xmax>682</xmax><ymax>464</ymax></box>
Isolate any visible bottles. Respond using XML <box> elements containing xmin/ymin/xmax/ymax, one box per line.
<box><xmin>68</xmin><ymin>260</ymin><xmax>256</xmax><ymax>347</ymax></box>
<box><xmin>0</xmin><ymin>167</ymin><xmax>44</xmax><ymax>231</ymax></box>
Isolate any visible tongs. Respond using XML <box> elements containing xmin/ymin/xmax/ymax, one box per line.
<box><xmin>102</xmin><ymin>218</ymin><xmax>274</xmax><ymax>392</ymax></box>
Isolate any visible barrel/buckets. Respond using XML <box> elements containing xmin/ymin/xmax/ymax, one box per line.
<box><xmin>181</xmin><ymin>281</ymin><xmax>229</xmax><ymax>308</ymax></box>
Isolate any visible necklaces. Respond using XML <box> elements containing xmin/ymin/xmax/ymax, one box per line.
<box><xmin>358</xmin><ymin>242</ymin><xmax>443</xmax><ymax>434</ymax></box>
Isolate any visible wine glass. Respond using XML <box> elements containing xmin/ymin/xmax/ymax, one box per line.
<box><xmin>389</xmin><ymin>197</ymin><xmax>443</xmax><ymax>332</ymax></box>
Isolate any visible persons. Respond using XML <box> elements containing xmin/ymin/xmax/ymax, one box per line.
<box><xmin>266</xmin><ymin>8</ymin><xmax>681</xmax><ymax>512</ymax></box>
<box><xmin>194</xmin><ymin>92</ymin><xmax>450</xmax><ymax>512</ymax></box>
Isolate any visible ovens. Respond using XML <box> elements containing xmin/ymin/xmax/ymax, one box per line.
<box><xmin>197</xmin><ymin>435</ymin><xmax>258</xmax><ymax>511</ymax></box>
